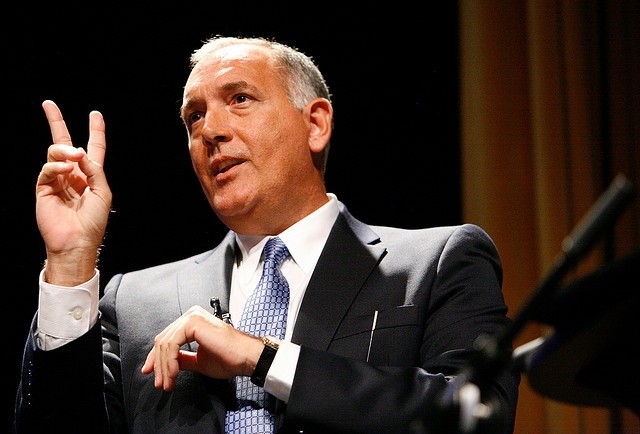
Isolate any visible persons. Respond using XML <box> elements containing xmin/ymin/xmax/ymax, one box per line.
<box><xmin>14</xmin><ymin>34</ymin><xmax>520</xmax><ymax>434</ymax></box>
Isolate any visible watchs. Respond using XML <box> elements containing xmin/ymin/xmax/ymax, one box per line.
<box><xmin>250</xmin><ymin>335</ymin><xmax>281</xmax><ymax>388</ymax></box>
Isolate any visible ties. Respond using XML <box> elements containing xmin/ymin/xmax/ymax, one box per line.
<box><xmin>224</xmin><ymin>237</ymin><xmax>291</xmax><ymax>434</ymax></box>
